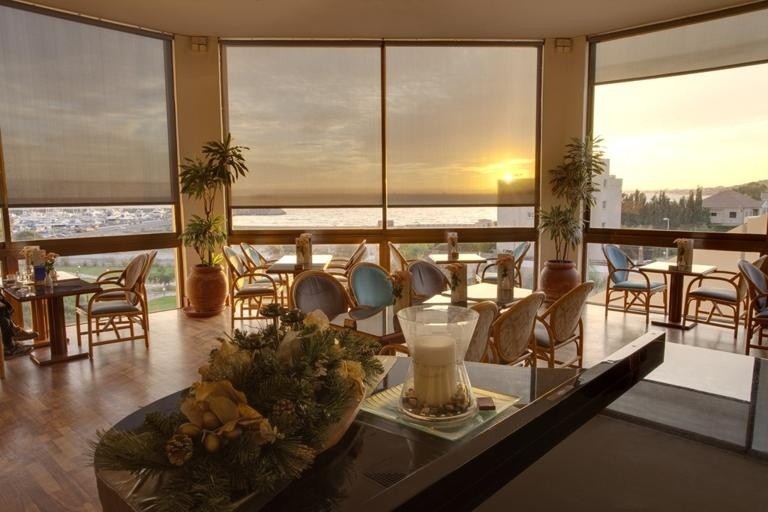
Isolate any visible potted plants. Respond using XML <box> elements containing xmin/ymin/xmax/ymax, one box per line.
<box><xmin>177</xmin><ymin>134</ymin><xmax>248</xmax><ymax>315</ymax></box>
<box><xmin>537</xmin><ymin>129</ymin><xmax>607</xmax><ymax>312</ymax></box>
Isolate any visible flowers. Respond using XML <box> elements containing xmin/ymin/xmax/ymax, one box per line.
<box><xmin>85</xmin><ymin>304</ymin><xmax>385</xmax><ymax>512</ymax></box>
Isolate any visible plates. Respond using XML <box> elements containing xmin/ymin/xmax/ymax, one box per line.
<box><xmin>361</xmin><ymin>381</ymin><xmax>521</xmax><ymax>440</ymax></box>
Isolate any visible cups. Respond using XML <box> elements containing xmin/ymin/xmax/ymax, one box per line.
<box><xmin>17</xmin><ymin>272</ymin><xmax>29</xmax><ymax>282</ymax></box>
<box><xmin>7</xmin><ymin>275</ymin><xmax>15</xmax><ymax>280</ymax></box>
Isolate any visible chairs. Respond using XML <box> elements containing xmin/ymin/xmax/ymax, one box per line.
<box><xmin>76</xmin><ymin>253</ymin><xmax>151</xmax><ymax>359</ymax></box>
<box><xmin>239</xmin><ymin>241</ymin><xmax>292</xmax><ymax>313</ymax></box>
<box><xmin>388</xmin><ymin>240</ymin><xmax>436</xmax><ymax>272</ymax></box>
<box><xmin>481</xmin><ymin>291</ymin><xmax>547</xmax><ymax>369</ymax></box>
<box><xmin>498</xmin><ymin>279</ymin><xmax>595</xmax><ymax>370</ymax></box>
<box><xmin>348</xmin><ymin>261</ymin><xmax>397</xmax><ymax>313</ymax></box>
<box><xmin>476</xmin><ymin>241</ymin><xmax>531</xmax><ymax>288</ymax></box>
<box><xmin>378</xmin><ymin>300</ymin><xmax>500</xmax><ymax>362</ymax></box>
<box><xmin>684</xmin><ymin>254</ymin><xmax>767</xmax><ymax>342</ymax></box>
<box><xmin>289</xmin><ymin>269</ymin><xmax>358</xmax><ymax>326</ymax></box>
<box><xmin>464</xmin><ymin>301</ymin><xmax>499</xmax><ymax>364</ymax></box>
<box><xmin>222</xmin><ymin>247</ymin><xmax>283</xmax><ymax>336</ymax></box>
<box><xmin>321</xmin><ymin>244</ymin><xmax>367</xmax><ymax>275</ymax></box>
<box><xmin>738</xmin><ymin>260</ymin><xmax>767</xmax><ymax>359</ymax></box>
<box><xmin>601</xmin><ymin>243</ymin><xmax>669</xmax><ymax>326</ymax></box>
<box><xmin>406</xmin><ymin>259</ymin><xmax>451</xmax><ymax>306</ymax></box>
<box><xmin>327</xmin><ymin>237</ymin><xmax>368</xmax><ymax>266</ymax></box>
<box><xmin>95</xmin><ymin>251</ymin><xmax>158</xmax><ymax>336</ymax></box>
